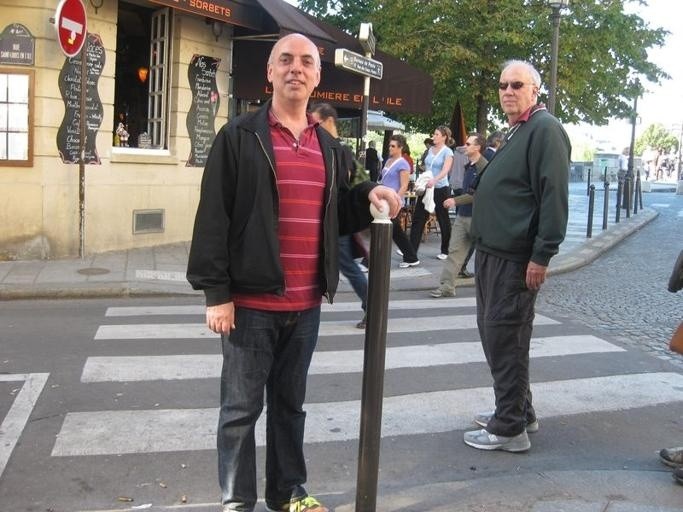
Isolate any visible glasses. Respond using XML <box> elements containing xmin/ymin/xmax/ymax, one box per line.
<box><xmin>499</xmin><ymin>81</ymin><xmax>523</xmax><ymax>90</ymax></box>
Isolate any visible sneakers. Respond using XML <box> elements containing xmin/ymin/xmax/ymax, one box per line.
<box><xmin>357</xmin><ymin>262</ymin><xmax>370</xmax><ymax>273</ymax></box>
<box><xmin>660</xmin><ymin>447</ymin><xmax>683</xmax><ymax>482</ymax></box>
<box><xmin>431</xmin><ymin>286</ymin><xmax>456</xmax><ymax>297</ymax></box>
<box><xmin>463</xmin><ymin>428</ymin><xmax>528</xmax><ymax>452</ymax></box>
<box><xmin>456</xmin><ymin>269</ymin><xmax>475</xmax><ymax>278</ymax></box>
<box><xmin>400</xmin><ymin>259</ymin><xmax>419</xmax><ymax>268</ymax></box>
<box><xmin>476</xmin><ymin>413</ymin><xmax>539</xmax><ymax>432</ymax></box>
<box><xmin>264</xmin><ymin>495</ymin><xmax>328</xmax><ymax>512</ymax></box>
<box><xmin>437</xmin><ymin>254</ymin><xmax>448</xmax><ymax>260</ymax></box>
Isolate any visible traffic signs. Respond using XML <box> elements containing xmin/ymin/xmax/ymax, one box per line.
<box><xmin>358</xmin><ymin>22</ymin><xmax>378</xmax><ymax>56</ymax></box>
<box><xmin>335</xmin><ymin>49</ymin><xmax>385</xmax><ymax>81</ymax></box>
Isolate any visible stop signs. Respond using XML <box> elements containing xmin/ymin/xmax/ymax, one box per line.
<box><xmin>54</xmin><ymin>0</ymin><xmax>87</xmax><ymax>57</ymax></box>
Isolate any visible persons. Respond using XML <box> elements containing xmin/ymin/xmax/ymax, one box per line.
<box><xmin>184</xmin><ymin>28</ymin><xmax>403</xmax><ymax>512</ymax></box>
<box><xmin>654</xmin><ymin>250</ymin><xmax>683</xmax><ymax>489</ymax></box>
<box><xmin>461</xmin><ymin>58</ymin><xmax>576</xmax><ymax>454</ymax></box>
<box><xmin>313</xmin><ymin>105</ymin><xmax>505</xmax><ymax>332</ymax></box>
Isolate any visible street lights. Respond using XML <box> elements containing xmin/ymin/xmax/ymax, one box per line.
<box><xmin>543</xmin><ymin>0</ymin><xmax>568</xmax><ymax>118</ymax></box>
<box><xmin>619</xmin><ymin>71</ymin><xmax>642</xmax><ymax>211</ymax></box>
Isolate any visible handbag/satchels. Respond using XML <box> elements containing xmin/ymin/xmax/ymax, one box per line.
<box><xmin>415</xmin><ymin>176</ymin><xmax>427</xmax><ymax>196</ymax></box>
